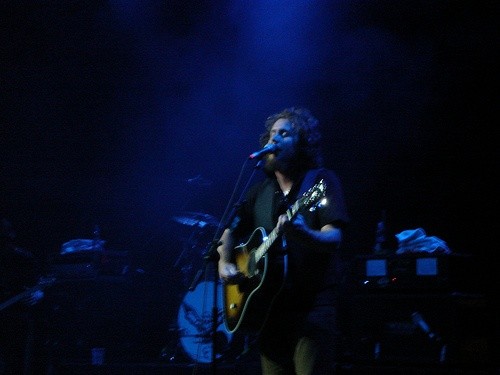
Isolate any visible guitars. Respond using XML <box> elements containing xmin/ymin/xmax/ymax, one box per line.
<box><xmin>223</xmin><ymin>179</ymin><xmax>327</xmax><ymax>333</ymax></box>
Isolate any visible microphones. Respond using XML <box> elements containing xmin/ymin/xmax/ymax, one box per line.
<box><xmin>248</xmin><ymin>142</ymin><xmax>286</xmax><ymax>161</ymax></box>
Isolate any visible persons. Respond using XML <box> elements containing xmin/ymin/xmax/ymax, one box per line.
<box><xmin>216</xmin><ymin>104</ymin><xmax>349</xmax><ymax>375</ymax></box>
